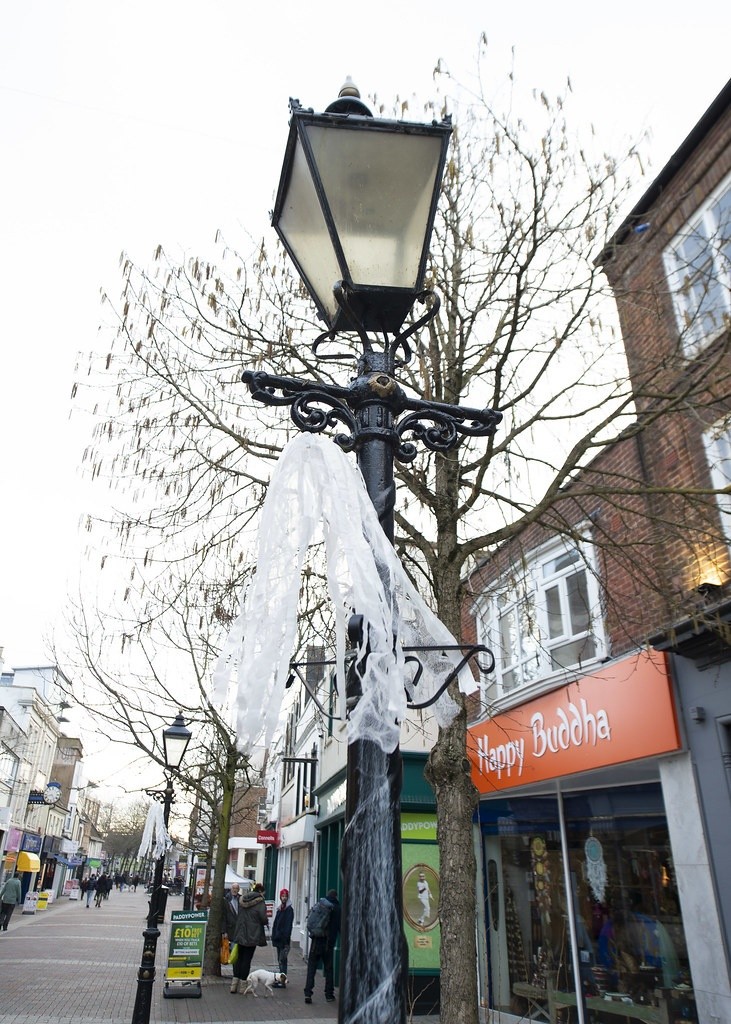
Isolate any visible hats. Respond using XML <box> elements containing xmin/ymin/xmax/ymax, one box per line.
<box><xmin>280</xmin><ymin>889</ymin><xmax>288</xmax><ymax>898</ymax></box>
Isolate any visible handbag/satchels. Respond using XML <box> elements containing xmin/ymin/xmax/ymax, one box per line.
<box><xmin>228</xmin><ymin>944</ymin><xmax>238</xmax><ymax>964</ymax></box>
<box><xmin>220</xmin><ymin>934</ymin><xmax>229</xmax><ymax>965</ymax></box>
<box><xmin>257</xmin><ymin>927</ymin><xmax>267</xmax><ymax>946</ymax></box>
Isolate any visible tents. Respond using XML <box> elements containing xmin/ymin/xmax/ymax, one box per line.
<box><xmin>211</xmin><ymin>863</ymin><xmax>254</xmax><ymax>889</ymax></box>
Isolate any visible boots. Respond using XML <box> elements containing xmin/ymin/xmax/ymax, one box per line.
<box><xmin>230</xmin><ymin>977</ymin><xmax>239</xmax><ymax>993</ymax></box>
<box><xmin>239</xmin><ymin>979</ymin><xmax>247</xmax><ymax>993</ymax></box>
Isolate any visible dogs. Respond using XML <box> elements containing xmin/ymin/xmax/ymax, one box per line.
<box><xmin>242</xmin><ymin>968</ymin><xmax>289</xmax><ymax>998</ymax></box>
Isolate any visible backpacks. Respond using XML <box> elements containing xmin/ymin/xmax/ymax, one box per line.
<box><xmin>306</xmin><ymin>899</ymin><xmax>337</xmax><ymax>937</ymax></box>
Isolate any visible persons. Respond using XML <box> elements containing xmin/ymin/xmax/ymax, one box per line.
<box><xmin>80</xmin><ymin>871</ymin><xmax>139</xmax><ymax>909</ymax></box>
<box><xmin>0</xmin><ymin>872</ymin><xmax>21</xmax><ymax>931</ymax></box>
<box><xmin>163</xmin><ymin>873</ymin><xmax>183</xmax><ymax>896</ymax></box>
<box><xmin>221</xmin><ymin>883</ymin><xmax>269</xmax><ymax>994</ymax></box>
<box><xmin>272</xmin><ymin>889</ymin><xmax>294</xmax><ymax>988</ymax></box>
<box><xmin>304</xmin><ymin>889</ymin><xmax>341</xmax><ymax>1004</ymax></box>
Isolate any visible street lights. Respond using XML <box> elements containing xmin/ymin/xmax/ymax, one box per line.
<box><xmin>244</xmin><ymin>72</ymin><xmax>502</xmax><ymax>1024</ymax></box>
<box><xmin>130</xmin><ymin>709</ymin><xmax>193</xmax><ymax>1023</ymax></box>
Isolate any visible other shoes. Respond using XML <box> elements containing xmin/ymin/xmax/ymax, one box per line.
<box><xmin>305</xmin><ymin>998</ymin><xmax>312</xmax><ymax>1004</ymax></box>
<box><xmin>325</xmin><ymin>994</ymin><xmax>335</xmax><ymax>1001</ymax></box>
<box><xmin>272</xmin><ymin>982</ymin><xmax>286</xmax><ymax>988</ymax></box>
<box><xmin>3</xmin><ymin>927</ymin><xmax>7</xmax><ymax>931</ymax></box>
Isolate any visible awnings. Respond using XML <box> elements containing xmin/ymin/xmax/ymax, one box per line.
<box><xmin>17</xmin><ymin>850</ymin><xmax>40</xmax><ymax>873</ymax></box>
<box><xmin>50</xmin><ymin>853</ymin><xmax>76</xmax><ymax>870</ymax></box>
